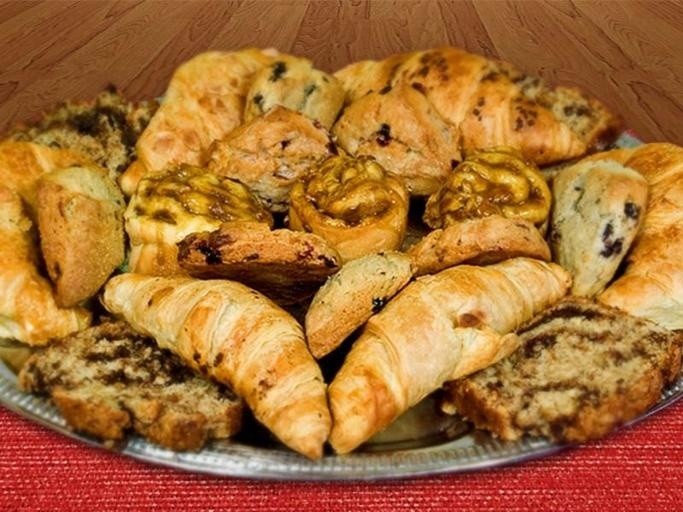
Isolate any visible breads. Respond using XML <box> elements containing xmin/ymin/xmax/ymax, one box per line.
<box><xmin>0</xmin><ymin>47</ymin><xmax>683</xmax><ymax>463</ymax></box>
